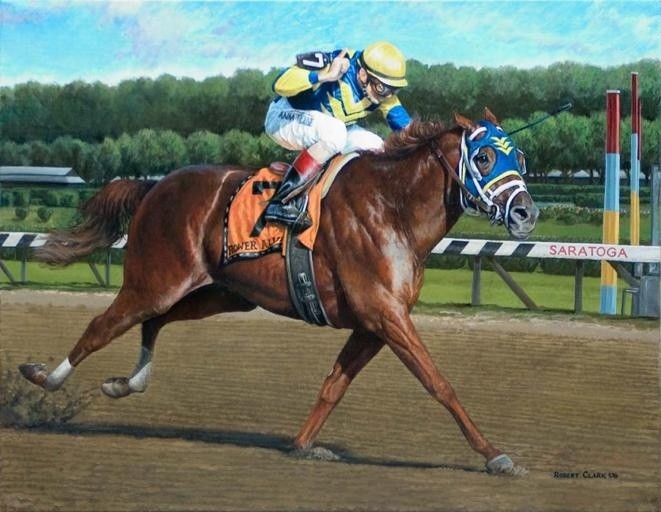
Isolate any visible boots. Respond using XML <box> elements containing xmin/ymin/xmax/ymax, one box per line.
<box><xmin>264</xmin><ymin>149</ymin><xmax>324</xmax><ymax>227</ymax></box>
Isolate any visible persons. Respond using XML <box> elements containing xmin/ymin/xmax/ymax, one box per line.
<box><xmin>264</xmin><ymin>41</ymin><xmax>413</xmax><ymax>231</ymax></box>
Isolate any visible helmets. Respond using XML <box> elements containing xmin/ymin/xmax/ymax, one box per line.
<box><xmin>356</xmin><ymin>41</ymin><xmax>408</xmax><ymax>87</ymax></box>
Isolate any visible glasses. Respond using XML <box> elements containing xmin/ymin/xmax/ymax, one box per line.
<box><xmin>369</xmin><ymin>78</ymin><xmax>403</xmax><ymax>96</ymax></box>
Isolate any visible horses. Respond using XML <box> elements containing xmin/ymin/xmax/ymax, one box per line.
<box><xmin>16</xmin><ymin>105</ymin><xmax>540</xmax><ymax>478</ymax></box>
